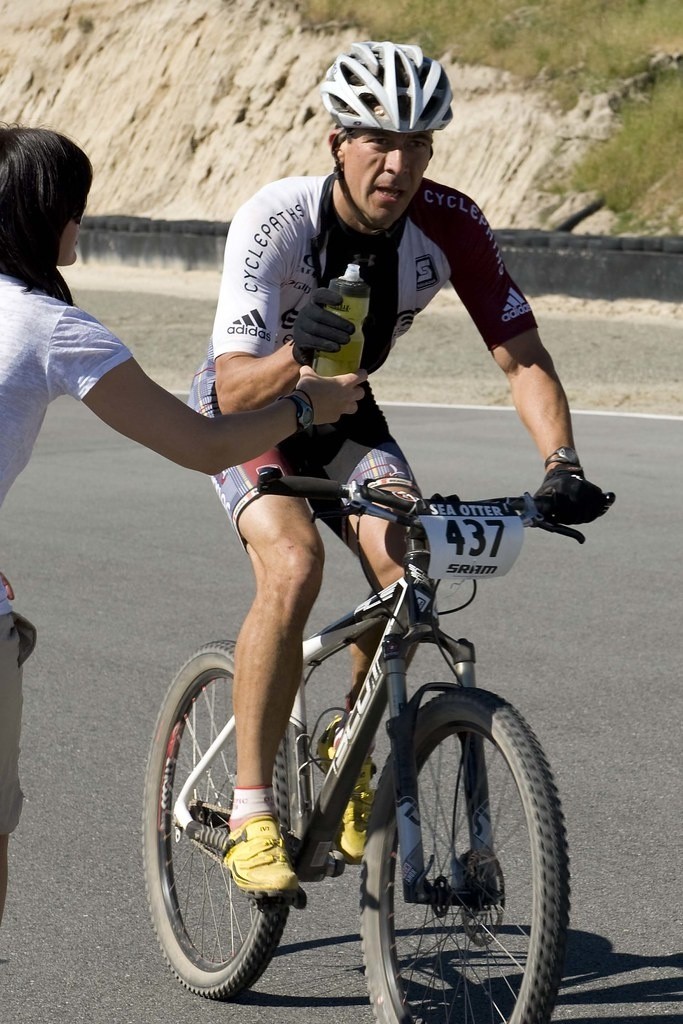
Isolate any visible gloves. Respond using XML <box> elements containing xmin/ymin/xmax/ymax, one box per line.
<box><xmin>534</xmin><ymin>462</ymin><xmax>604</xmax><ymax>525</ymax></box>
<box><xmin>291</xmin><ymin>287</ymin><xmax>356</xmax><ymax>369</ymax></box>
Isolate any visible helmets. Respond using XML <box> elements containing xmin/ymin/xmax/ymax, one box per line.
<box><xmin>321</xmin><ymin>40</ymin><xmax>453</xmax><ymax>133</ymax></box>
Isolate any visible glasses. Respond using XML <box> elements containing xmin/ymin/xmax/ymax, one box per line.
<box><xmin>71</xmin><ymin>202</ymin><xmax>87</xmax><ymax>225</ymax></box>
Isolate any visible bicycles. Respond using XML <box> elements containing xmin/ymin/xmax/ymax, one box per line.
<box><xmin>139</xmin><ymin>465</ymin><xmax>618</xmax><ymax>1024</ymax></box>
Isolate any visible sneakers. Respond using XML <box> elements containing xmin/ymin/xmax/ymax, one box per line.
<box><xmin>219</xmin><ymin>815</ymin><xmax>299</xmax><ymax>892</ymax></box>
<box><xmin>316</xmin><ymin>714</ymin><xmax>377</xmax><ymax>865</ymax></box>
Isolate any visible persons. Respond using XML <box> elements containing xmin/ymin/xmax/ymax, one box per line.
<box><xmin>0</xmin><ymin>125</ymin><xmax>368</xmax><ymax>921</ymax></box>
<box><xmin>191</xmin><ymin>42</ymin><xmax>603</xmax><ymax>895</ymax></box>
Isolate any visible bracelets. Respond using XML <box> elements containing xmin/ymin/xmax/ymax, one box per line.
<box><xmin>292</xmin><ymin>389</ymin><xmax>314</xmax><ymax>412</ymax></box>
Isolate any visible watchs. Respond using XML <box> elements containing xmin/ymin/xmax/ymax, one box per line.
<box><xmin>277</xmin><ymin>395</ymin><xmax>314</xmax><ymax>434</ymax></box>
<box><xmin>545</xmin><ymin>447</ymin><xmax>580</xmax><ymax>469</ymax></box>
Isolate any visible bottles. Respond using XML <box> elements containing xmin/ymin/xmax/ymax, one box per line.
<box><xmin>312</xmin><ymin>263</ymin><xmax>369</xmax><ymax>376</ymax></box>
